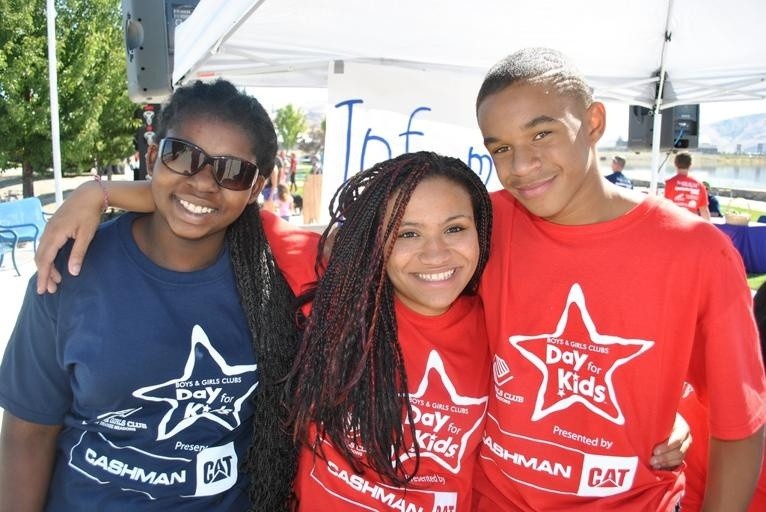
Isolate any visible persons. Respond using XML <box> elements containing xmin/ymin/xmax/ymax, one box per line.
<box><xmin>0</xmin><ymin>75</ymin><xmax>312</xmax><ymax>512</ymax></box>
<box><xmin>700</xmin><ymin>178</ymin><xmax>722</xmax><ymax>216</ymax></box>
<box><xmin>264</xmin><ymin>145</ymin><xmax>323</xmax><ymax>218</ymax></box>
<box><xmin>663</xmin><ymin>151</ymin><xmax>712</xmax><ymax>220</ymax></box>
<box><xmin>603</xmin><ymin>155</ymin><xmax>634</xmax><ymax>190</ymax></box>
<box><xmin>320</xmin><ymin>44</ymin><xmax>766</xmax><ymax>510</ymax></box>
<box><xmin>32</xmin><ymin>149</ymin><xmax>699</xmax><ymax>510</ymax></box>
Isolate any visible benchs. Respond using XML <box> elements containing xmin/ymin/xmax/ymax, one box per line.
<box><xmin>0</xmin><ymin>197</ymin><xmax>54</xmax><ymax>277</ymax></box>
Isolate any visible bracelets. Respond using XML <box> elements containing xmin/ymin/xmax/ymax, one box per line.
<box><xmin>93</xmin><ymin>175</ymin><xmax>111</xmax><ymax>209</ymax></box>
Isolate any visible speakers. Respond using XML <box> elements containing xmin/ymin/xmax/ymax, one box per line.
<box><xmin>629</xmin><ymin>103</ymin><xmax>701</xmax><ymax>154</ymax></box>
<box><xmin>122</xmin><ymin>1</ymin><xmax>196</xmax><ymax>104</ymax></box>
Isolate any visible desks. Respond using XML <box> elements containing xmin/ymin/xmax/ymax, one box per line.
<box><xmin>709</xmin><ymin>217</ymin><xmax>765</xmax><ymax>274</ymax></box>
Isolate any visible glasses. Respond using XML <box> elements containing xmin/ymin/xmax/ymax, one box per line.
<box><xmin>158</xmin><ymin>136</ymin><xmax>260</xmax><ymax>192</ymax></box>
